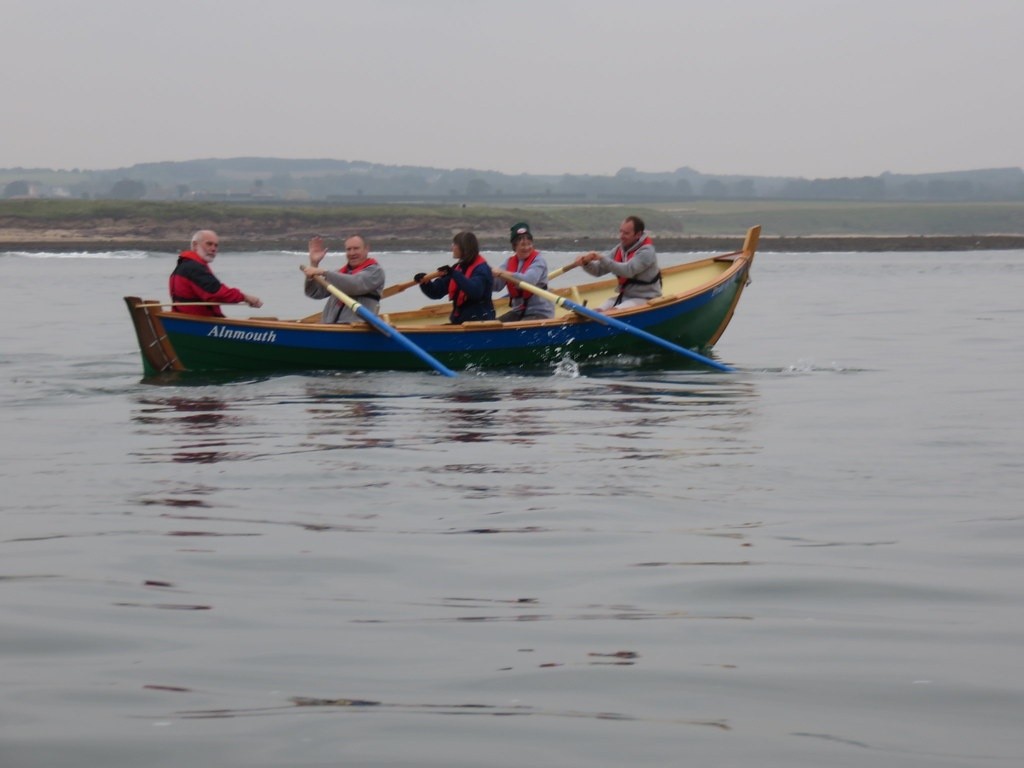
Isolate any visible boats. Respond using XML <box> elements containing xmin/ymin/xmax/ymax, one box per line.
<box><xmin>123</xmin><ymin>224</ymin><xmax>764</xmax><ymax>386</ymax></box>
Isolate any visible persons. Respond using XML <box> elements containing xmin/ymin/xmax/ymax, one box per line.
<box><xmin>414</xmin><ymin>231</ymin><xmax>497</xmax><ymax>325</ymax></box>
<box><xmin>169</xmin><ymin>230</ymin><xmax>263</xmax><ymax>318</ymax></box>
<box><xmin>575</xmin><ymin>214</ymin><xmax>662</xmax><ymax>312</ymax></box>
<box><xmin>305</xmin><ymin>236</ymin><xmax>385</xmax><ymax>324</ymax></box>
<box><xmin>490</xmin><ymin>223</ymin><xmax>555</xmax><ymax>323</ymax></box>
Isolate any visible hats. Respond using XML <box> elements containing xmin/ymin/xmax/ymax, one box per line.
<box><xmin>511</xmin><ymin>223</ymin><xmax>531</xmax><ymax>242</ymax></box>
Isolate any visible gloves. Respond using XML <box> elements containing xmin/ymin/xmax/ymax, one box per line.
<box><xmin>438</xmin><ymin>266</ymin><xmax>451</xmax><ymax>279</ymax></box>
<box><xmin>414</xmin><ymin>273</ymin><xmax>430</xmax><ymax>286</ymax></box>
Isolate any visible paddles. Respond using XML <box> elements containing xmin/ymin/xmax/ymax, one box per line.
<box><xmin>299</xmin><ymin>264</ymin><xmax>458</xmax><ymax>380</ymax></box>
<box><xmin>547</xmin><ymin>253</ymin><xmax>596</xmax><ymax>282</ymax></box>
<box><xmin>303</xmin><ymin>269</ymin><xmax>447</xmax><ymax>323</ymax></box>
<box><xmin>493</xmin><ymin>269</ymin><xmax>734</xmax><ymax>373</ymax></box>
